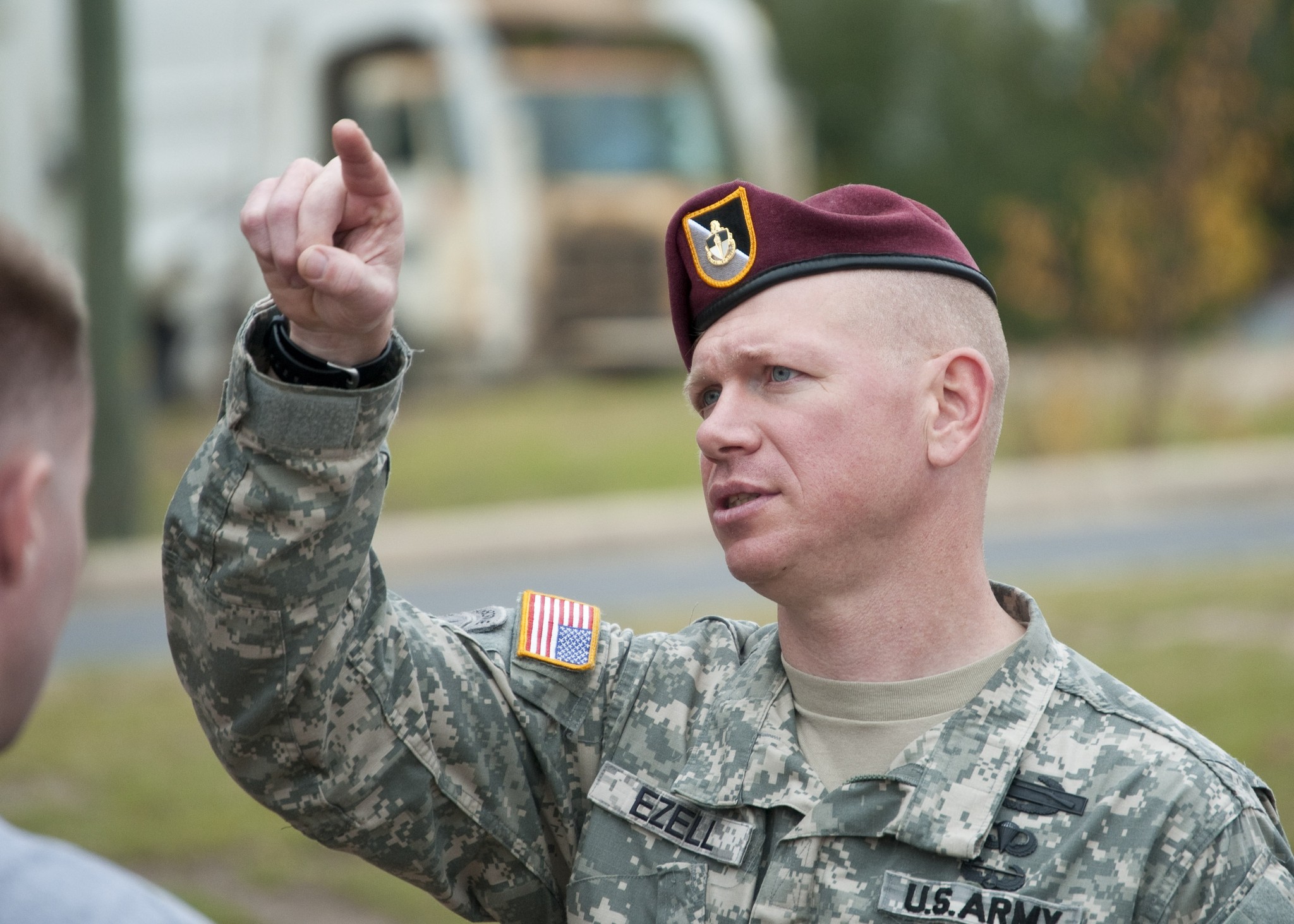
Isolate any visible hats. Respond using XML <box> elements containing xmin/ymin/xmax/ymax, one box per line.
<box><xmin>665</xmin><ymin>180</ymin><xmax>999</xmax><ymax>373</ymax></box>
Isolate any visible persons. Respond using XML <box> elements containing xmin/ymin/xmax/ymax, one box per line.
<box><xmin>0</xmin><ymin>206</ymin><xmax>230</xmax><ymax>924</ymax></box>
<box><xmin>161</xmin><ymin>115</ymin><xmax>1293</xmax><ymax>924</ymax></box>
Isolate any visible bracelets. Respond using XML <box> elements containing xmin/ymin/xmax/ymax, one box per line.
<box><xmin>264</xmin><ymin>307</ymin><xmax>395</xmax><ymax>389</ymax></box>
